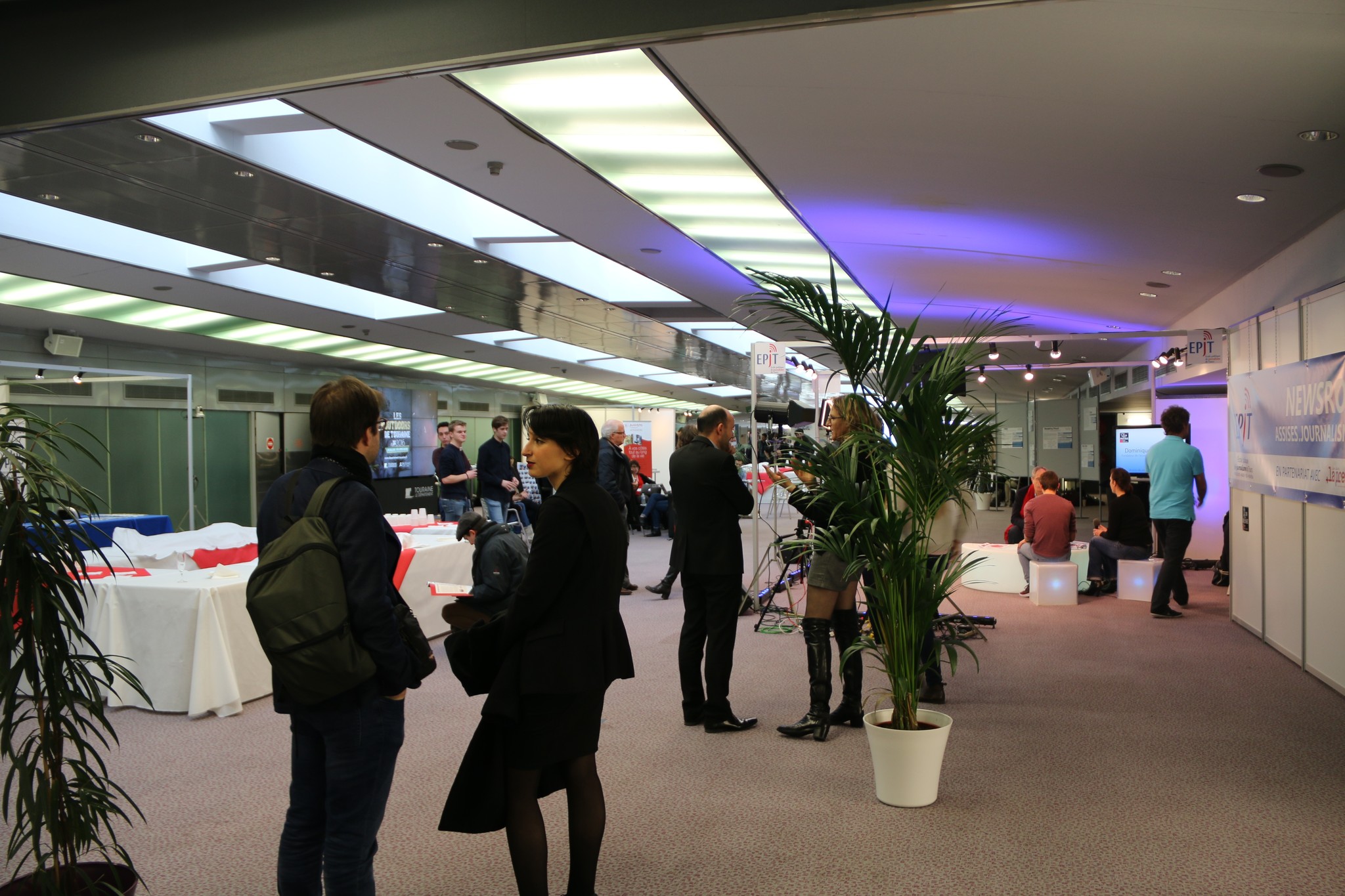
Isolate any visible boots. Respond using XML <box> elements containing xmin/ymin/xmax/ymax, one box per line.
<box><xmin>777</xmin><ymin>618</ymin><xmax>832</xmax><ymax>740</ymax></box>
<box><xmin>830</xmin><ymin>609</ymin><xmax>864</xmax><ymax>727</ymax></box>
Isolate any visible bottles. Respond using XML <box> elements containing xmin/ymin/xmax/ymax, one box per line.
<box><xmin>641</xmin><ymin>493</ymin><xmax>646</xmax><ymax>505</ymax></box>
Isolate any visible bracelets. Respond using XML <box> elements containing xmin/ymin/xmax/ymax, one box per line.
<box><xmin>466</xmin><ymin>473</ymin><xmax>468</xmax><ymax>479</ymax></box>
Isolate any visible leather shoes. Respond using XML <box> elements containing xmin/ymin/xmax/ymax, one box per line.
<box><xmin>704</xmin><ymin>713</ymin><xmax>758</xmax><ymax>733</ymax></box>
<box><xmin>684</xmin><ymin>714</ymin><xmax>705</xmax><ymax>725</ymax></box>
<box><xmin>916</xmin><ymin>682</ymin><xmax>947</xmax><ymax>704</ymax></box>
<box><xmin>916</xmin><ymin>675</ymin><xmax>926</xmax><ymax>688</ymax></box>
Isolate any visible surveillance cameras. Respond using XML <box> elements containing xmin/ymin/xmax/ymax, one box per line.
<box><xmin>708</xmin><ymin>384</ymin><xmax>714</xmax><ymax>386</ymax></box>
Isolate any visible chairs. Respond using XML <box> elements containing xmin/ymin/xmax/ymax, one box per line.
<box><xmin>767</xmin><ymin>479</ymin><xmax>804</xmax><ymax>520</ymax></box>
<box><xmin>480</xmin><ymin>498</ymin><xmax>531</xmax><ymax>549</ymax></box>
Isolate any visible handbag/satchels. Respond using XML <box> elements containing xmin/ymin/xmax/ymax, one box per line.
<box><xmin>1212</xmin><ymin>560</ymin><xmax>1230</xmax><ymax>586</ymax></box>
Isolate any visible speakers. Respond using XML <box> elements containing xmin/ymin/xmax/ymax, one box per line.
<box><xmin>43</xmin><ymin>334</ymin><xmax>83</xmax><ymax>358</ymax></box>
<box><xmin>1087</xmin><ymin>368</ymin><xmax>1108</xmax><ymax>388</ymax></box>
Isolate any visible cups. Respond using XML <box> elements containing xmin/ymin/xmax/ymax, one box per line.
<box><xmin>784</xmin><ymin>460</ymin><xmax>789</xmax><ymax>468</ymax></box>
<box><xmin>470</xmin><ymin>467</ymin><xmax>476</xmax><ymax>470</ymax></box>
<box><xmin>382</xmin><ymin>508</ymin><xmax>435</xmax><ymax>526</ymax></box>
<box><xmin>32</xmin><ymin>543</ymin><xmax>82</xmax><ymax>573</ymax></box>
<box><xmin>802</xmin><ymin>460</ymin><xmax>805</xmax><ymax>464</ymax></box>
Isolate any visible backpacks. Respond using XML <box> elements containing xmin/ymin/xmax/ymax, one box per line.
<box><xmin>246</xmin><ymin>476</ymin><xmax>376</xmax><ymax>712</ymax></box>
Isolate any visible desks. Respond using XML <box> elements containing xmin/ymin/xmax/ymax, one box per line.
<box><xmin>960</xmin><ymin>540</ymin><xmax>1091</xmax><ymax>593</ymax></box>
<box><xmin>742</xmin><ymin>479</ymin><xmax>765</xmax><ymax>519</ymax></box>
<box><xmin>0</xmin><ymin>513</ymin><xmax>474</xmax><ymax>721</ymax></box>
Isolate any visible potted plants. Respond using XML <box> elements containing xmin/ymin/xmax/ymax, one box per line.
<box><xmin>0</xmin><ymin>388</ymin><xmax>149</xmax><ymax>895</ymax></box>
<box><xmin>732</xmin><ymin>255</ymin><xmax>1040</xmax><ymax>812</ymax></box>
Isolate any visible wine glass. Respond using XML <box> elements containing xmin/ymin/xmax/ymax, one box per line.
<box><xmin>176</xmin><ymin>552</ymin><xmax>187</xmax><ymax>583</ymax></box>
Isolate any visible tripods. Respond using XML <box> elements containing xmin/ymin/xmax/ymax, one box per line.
<box><xmin>736</xmin><ymin>421</ymin><xmax>794</xmax><ymax>616</ymax></box>
<box><xmin>752</xmin><ymin>528</ymin><xmax>811</xmax><ymax>634</ymax></box>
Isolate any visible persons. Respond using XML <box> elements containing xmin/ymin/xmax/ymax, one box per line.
<box><xmin>1146</xmin><ymin>406</ymin><xmax>1207</xmax><ymax>617</ymax></box>
<box><xmin>734</xmin><ymin>393</ymin><xmax>978</xmax><ymax>742</ymax></box>
<box><xmin>670</xmin><ymin>405</ymin><xmax>759</xmax><ymax>734</ymax></box>
<box><xmin>1083</xmin><ymin>467</ymin><xmax>1154</xmax><ymax>596</ymax></box>
<box><xmin>1017</xmin><ymin>470</ymin><xmax>1077</xmax><ymax>597</ymax></box>
<box><xmin>432</xmin><ymin>415</ymin><xmax>697</xmax><ymax>630</ymax></box>
<box><xmin>435</xmin><ymin>403</ymin><xmax>634</xmax><ymax>896</ymax></box>
<box><xmin>1008</xmin><ymin>466</ymin><xmax>1047</xmax><ymax>544</ymax></box>
<box><xmin>257</xmin><ymin>374</ymin><xmax>436</xmax><ymax>896</ymax></box>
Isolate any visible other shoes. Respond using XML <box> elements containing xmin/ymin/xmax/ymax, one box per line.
<box><xmin>647</xmin><ymin>528</ymin><xmax>661</xmax><ymax>537</ymax></box>
<box><xmin>620</xmin><ymin>588</ymin><xmax>632</xmax><ymax>595</ymax></box>
<box><xmin>628</xmin><ymin>584</ymin><xmax>638</xmax><ymax>590</ymax></box>
<box><xmin>636</xmin><ymin>517</ymin><xmax>645</xmax><ymax>526</ymax></box>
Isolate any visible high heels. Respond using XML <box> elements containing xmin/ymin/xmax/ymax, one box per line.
<box><xmin>1101</xmin><ymin>580</ymin><xmax>1117</xmax><ymax>593</ymax></box>
<box><xmin>1080</xmin><ymin>580</ymin><xmax>1101</xmax><ymax>597</ymax></box>
<box><xmin>645</xmin><ymin>579</ymin><xmax>671</xmax><ymax>599</ymax></box>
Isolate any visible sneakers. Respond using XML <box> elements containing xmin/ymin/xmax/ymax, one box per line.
<box><xmin>1019</xmin><ymin>584</ymin><xmax>1030</xmax><ymax>597</ymax></box>
<box><xmin>1150</xmin><ymin>605</ymin><xmax>1183</xmax><ymax>618</ymax></box>
<box><xmin>1173</xmin><ymin>594</ymin><xmax>1188</xmax><ymax>608</ymax></box>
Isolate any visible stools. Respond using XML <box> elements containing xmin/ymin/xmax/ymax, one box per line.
<box><xmin>1028</xmin><ymin>559</ymin><xmax>1079</xmax><ymax>604</ymax></box>
<box><xmin>1116</xmin><ymin>555</ymin><xmax>1174</xmax><ymax>602</ymax></box>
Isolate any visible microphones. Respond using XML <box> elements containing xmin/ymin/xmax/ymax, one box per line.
<box><xmin>1093</xmin><ymin>518</ymin><xmax>1099</xmax><ymax>530</ymax></box>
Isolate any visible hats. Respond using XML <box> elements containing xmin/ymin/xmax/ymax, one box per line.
<box><xmin>456</xmin><ymin>511</ymin><xmax>481</xmax><ymax>541</ymax></box>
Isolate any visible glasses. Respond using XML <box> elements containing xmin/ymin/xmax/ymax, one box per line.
<box><xmin>613</xmin><ymin>432</ymin><xmax>625</xmax><ymax>436</ymax></box>
<box><xmin>828</xmin><ymin>416</ymin><xmax>844</xmax><ymax>423</ymax></box>
<box><xmin>631</xmin><ymin>466</ymin><xmax>638</xmax><ymax>468</ymax></box>
<box><xmin>376</xmin><ymin>418</ymin><xmax>387</xmax><ymax>432</ymax></box>
<box><xmin>465</xmin><ymin>535</ymin><xmax>469</xmax><ymax>543</ymax></box>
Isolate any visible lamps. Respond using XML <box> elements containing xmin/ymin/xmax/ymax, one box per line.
<box><xmin>1151</xmin><ymin>347</ymin><xmax>1183</xmax><ymax>370</ymax></box>
<box><xmin>977</xmin><ymin>364</ymin><xmax>986</xmax><ymax>382</ymax></box>
<box><xmin>988</xmin><ymin>341</ymin><xmax>1001</xmax><ymax>362</ymax></box>
<box><xmin>1048</xmin><ymin>341</ymin><xmax>1060</xmax><ymax>360</ymax></box>
<box><xmin>34</xmin><ymin>368</ymin><xmax>46</xmax><ymax>380</ymax></box>
<box><xmin>639</xmin><ymin>407</ymin><xmax>698</xmax><ymax>417</ymax></box>
<box><xmin>72</xmin><ymin>371</ymin><xmax>87</xmax><ymax>385</ymax></box>
<box><xmin>1023</xmin><ymin>363</ymin><xmax>1035</xmax><ymax>381</ymax></box>
